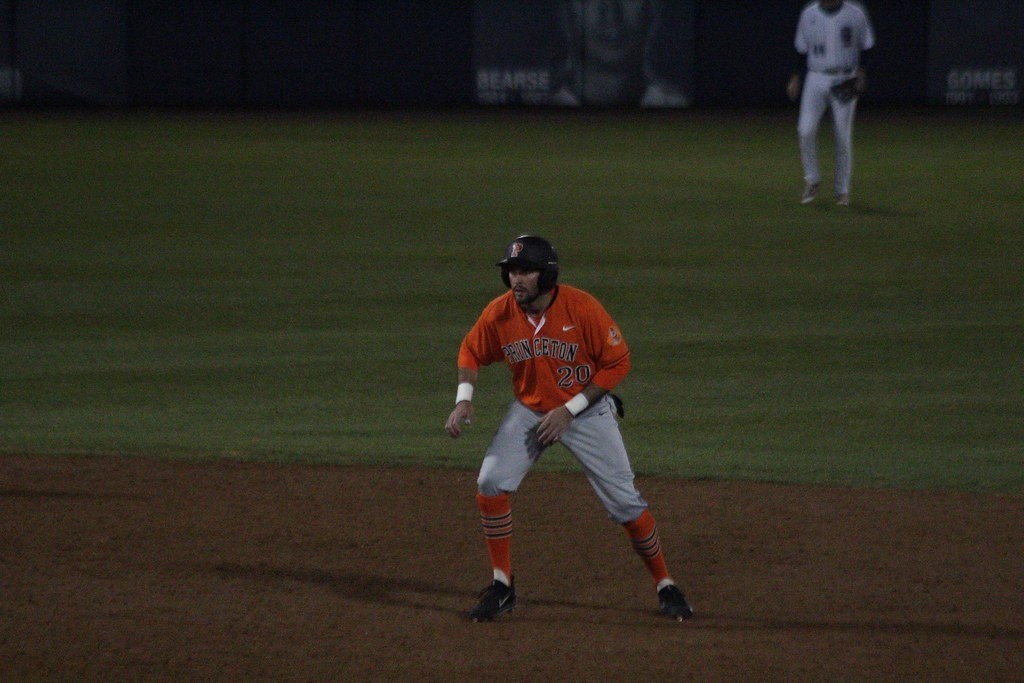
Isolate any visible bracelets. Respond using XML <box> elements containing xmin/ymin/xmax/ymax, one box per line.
<box><xmin>455</xmin><ymin>382</ymin><xmax>473</xmax><ymax>405</ymax></box>
<box><xmin>565</xmin><ymin>392</ymin><xmax>588</xmax><ymax>416</ymax></box>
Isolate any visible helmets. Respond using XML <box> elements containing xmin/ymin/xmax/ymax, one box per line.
<box><xmin>495</xmin><ymin>235</ymin><xmax>559</xmax><ymax>294</ymax></box>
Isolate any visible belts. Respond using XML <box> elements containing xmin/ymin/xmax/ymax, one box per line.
<box><xmin>810</xmin><ymin>67</ymin><xmax>852</xmax><ymax>74</ymax></box>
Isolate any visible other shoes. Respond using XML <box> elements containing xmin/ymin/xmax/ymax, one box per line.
<box><xmin>835</xmin><ymin>194</ymin><xmax>849</xmax><ymax>207</ymax></box>
<box><xmin>800</xmin><ymin>182</ymin><xmax>820</xmax><ymax>204</ymax></box>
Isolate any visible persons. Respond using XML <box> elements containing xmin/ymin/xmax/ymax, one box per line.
<box><xmin>787</xmin><ymin>0</ymin><xmax>876</xmax><ymax>206</ymax></box>
<box><xmin>446</xmin><ymin>235</ymin><xmax>693</xmax><ymax>623</ymax></box>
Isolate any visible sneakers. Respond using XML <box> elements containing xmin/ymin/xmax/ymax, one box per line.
<box><xmin>470</xmin><ymin>575</ymin><xmax>516</xmax><ymax>622</ymax></box>
<box><xmin>656</xmin><ymin>584</ymin><xmax>693</xmax><ymax>622</ymax></box>
